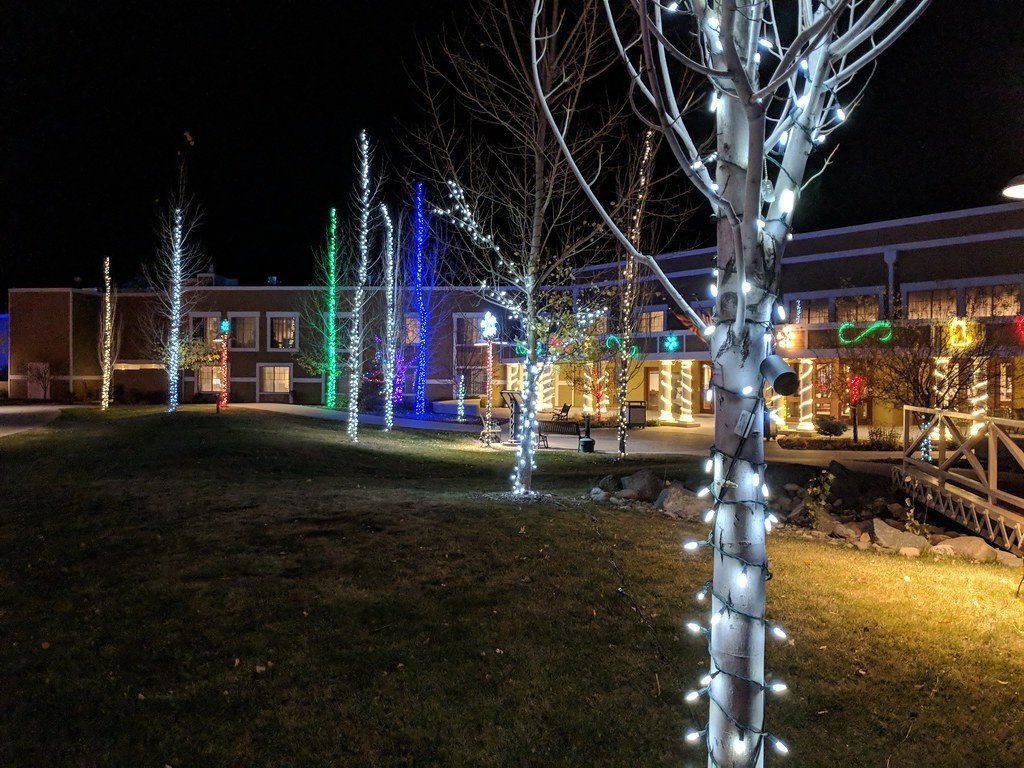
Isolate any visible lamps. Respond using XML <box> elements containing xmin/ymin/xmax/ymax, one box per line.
<box><xmin>760</xmin><ymin>354</ymin><xmax>800</xmax><ymax>396</ymax></box>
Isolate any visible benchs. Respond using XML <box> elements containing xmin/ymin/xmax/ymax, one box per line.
<box><xmin>537</xmin><ymin>419</ymin><xmax>587</xmax><ymax>452</ymax></box>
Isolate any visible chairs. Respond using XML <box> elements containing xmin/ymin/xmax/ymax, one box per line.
<box><xmin>551</xmin><ymin>403</ymin><xmax>571</xmax><ymax>420</ymax></box>
<box><xmin>480</xmin><ymin>414</ymin><xmax>502</xmax><ymax>443</ymax></box>
